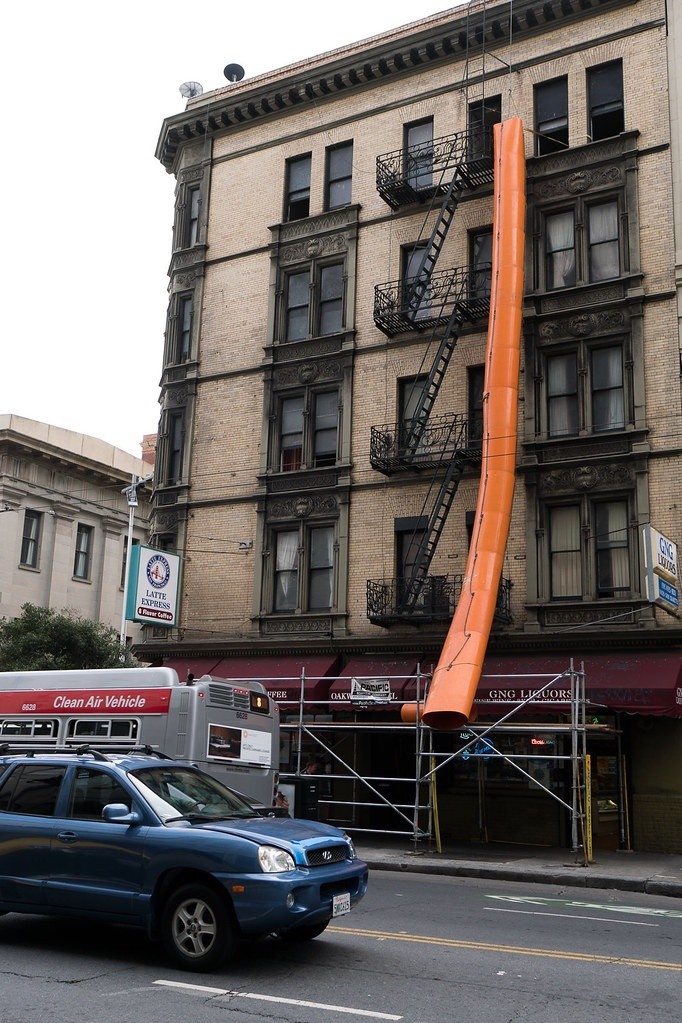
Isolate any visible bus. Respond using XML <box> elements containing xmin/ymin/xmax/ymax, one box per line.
<box><xmin>0</xmin><ymin>669</ymin><xmax>280</xmax><ymax>808</ymax></box>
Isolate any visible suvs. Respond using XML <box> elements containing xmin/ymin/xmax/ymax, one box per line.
<box><xmin>0</xmin><ymin>744</ymin><xmax>369</xmax><ymax>972</ymax></box>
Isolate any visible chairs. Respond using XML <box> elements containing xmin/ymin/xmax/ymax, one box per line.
<box><xmin>109</xmin><ymin>788</ymin><xmax>130</xmax><ymax>809</ymax></box>
<box><xmin>69</xmin><ymin>788</ymin><xmax>86</xmax><ymax>812</ymax></box>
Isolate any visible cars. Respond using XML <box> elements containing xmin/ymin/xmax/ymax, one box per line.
<box><xmin>163</xmin><ymin>773</ymin><xmax>291</xmax><ymax>818</ymax></box>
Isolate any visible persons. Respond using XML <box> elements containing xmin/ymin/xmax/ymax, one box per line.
<box><xmin>301</xmin><ymin>757</ymin><xmax>322</xmax><ymax>774</ymax></box>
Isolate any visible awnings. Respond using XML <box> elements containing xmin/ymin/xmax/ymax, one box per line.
<box><xmin>158</xmin><ymin>654</ymin><xmax>343</xmax><ymax>714</ymax></box>
<box><xmin>328</xmin><ymin>649</ymin><xmax>682</xmax><ymax>720</ymax></box>
